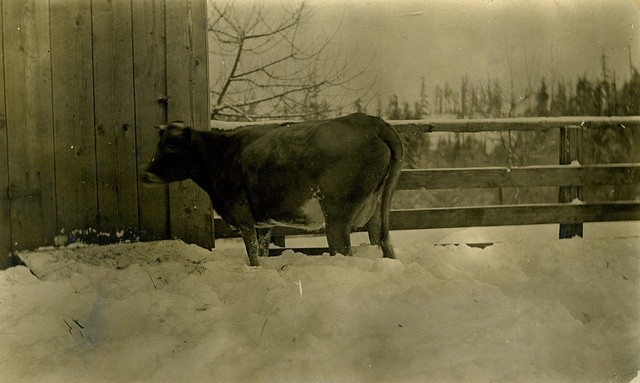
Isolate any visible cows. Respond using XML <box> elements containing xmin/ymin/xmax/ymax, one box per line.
<box><xmin>140</xmin><ymin>112</ymin><xmax>405</xmax><ymax>266</ymax></box>
